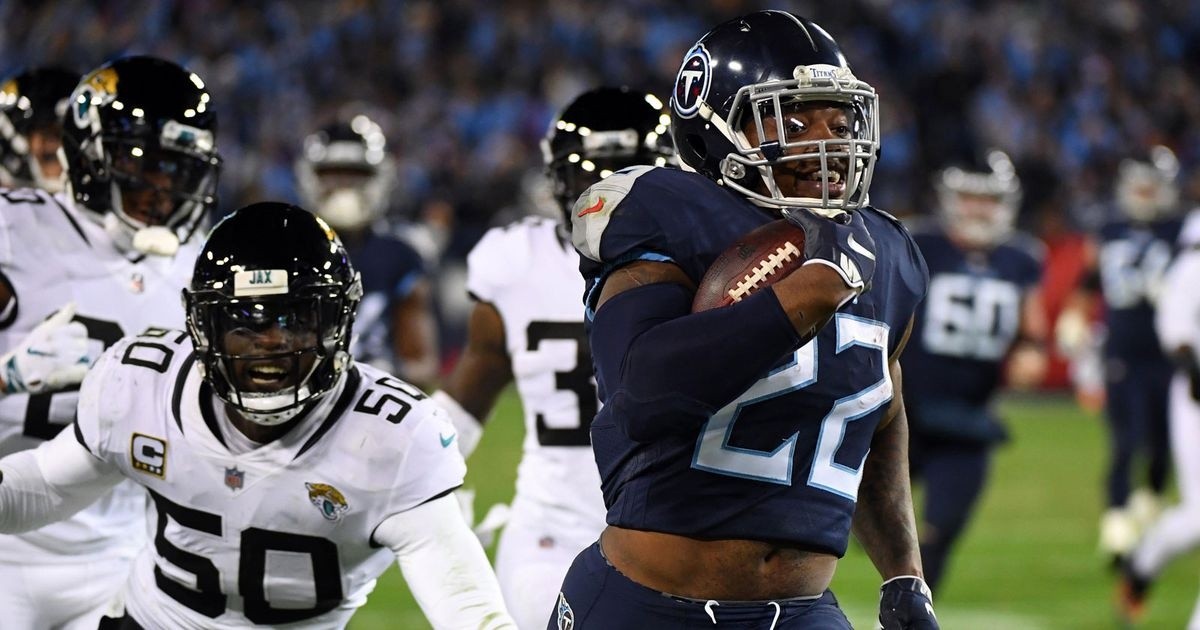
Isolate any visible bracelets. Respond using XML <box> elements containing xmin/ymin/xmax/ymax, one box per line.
<box><xmin>1019</xmin><ymin>330</ymin><xmax>1046</xmax><ymax>350</ymax></box>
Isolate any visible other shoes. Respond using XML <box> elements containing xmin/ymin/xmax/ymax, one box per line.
<box><xmin>1132</xmin><ymin>488</ymin><xmax>1155</xmax><ymax>531</ymax></box>
<box><xmin>1098</xmin><ymin>508</ymin><xmax>1132</xmax><ymax>568</ymax></box>
<box><xmin>1113</xmin><ymin>552</ymin><xmax>1151</xmax><ymax>626</ymax></box>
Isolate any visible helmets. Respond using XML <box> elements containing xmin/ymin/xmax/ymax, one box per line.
<box><xmin>295</xmin><ymin>118</ymin><xmax>396</xmax><ymax>227</ymax></box>
<box><xmin>0</xmin><ymin>67</ymin><xmax>84</xmax><ymax>187</ymax></box>
<box><xmin>180</xmin><ymin>202</ymin><xmax>366</xmax><ymax>425</ymax></box>
<box><xmin>1115</xmin><ymin>143</ymin><xmax>1180</xmax><ymax>219</ymax></box>
<box><xmin>670</xmin><ymin>8</ymin><xmax>881</xmax><ymax>216</ymax></box>
<box><xmin>540</xmin><ymin>89</ymin><xmax>676</xmax><ymax>232</ymax></box>
<box><xmin>62</xmin><ymin>56</ymin><xmax>223</xmax><ymax>247</ymax></box>
<box><xmin>939</xmin><ymin>147</ymin><xmax>1021</xmax><ymax>245</ymax></box>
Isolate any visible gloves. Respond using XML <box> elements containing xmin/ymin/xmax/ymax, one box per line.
<box><xmin>787</xmin><ymin>205</ymin><xmax>877</xmax><ymax>312</ymax></box>
<box><xmin>1</xmin><ymin>297</ymin><xmax>104</xmax><ymax>395</ymax></box>
<box><xmin>878</xmin><ymin>576</ymin><xmax>942</xmax><ymax>629</ymax></box>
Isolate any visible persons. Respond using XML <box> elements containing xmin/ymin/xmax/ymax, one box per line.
<box><xmin>3</xmin><ymin>1</ymin><xmax>1197</xmax><ymax>342</ymax></box>
<box><xmin>298</xmin><ymin>111</ymin><xmax>438</xmax><ymax>392</ymax></box>
<box><xmin>539</xmin><ymin>8</ymin><xmax>944</xmax><ymax>630</ymax></box>
<box><xmin>441</xmin><ymin>86</ymin><xmax>673</xmax><ymax>627</ymax></box>
<box><xmin>892</xmin><ymin>146</ymin><xmax>1050</xmax><ymax>592</ymax></box>
<box><xmin>0</xmin><ymin>200</ymin><xmax>519</xmax><ymax>630</ymax></box>
<box><xmin>0</xmin><ymin>55</ymin><xmax>221</xmax><ymax>627</ymax></box>
<box><xmin>1054</xmin><ymin>150</ymin><xmax>1199</xmax><ymax>615</ymax></box>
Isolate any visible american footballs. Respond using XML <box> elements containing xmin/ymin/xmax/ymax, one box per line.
<box><xmin>687</xmin><ymin>219</ymin><xmax>839</xmax><ymax>375</ymax></box>
